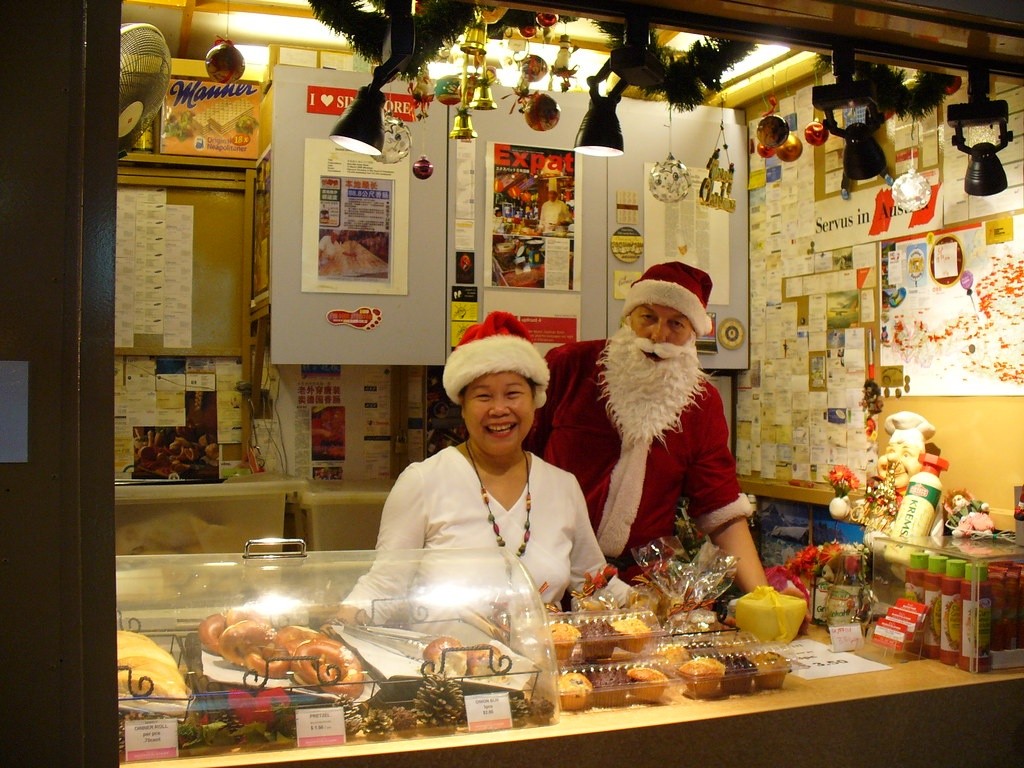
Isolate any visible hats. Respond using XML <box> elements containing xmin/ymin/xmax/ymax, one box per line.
<box><xmin>444</xmin><ymin>309</ymin><xmax>550</xmax><ymax>409</ymax></box>
<box><xmin>549</xmin><ymin>178</ymin><xmax>558</xmax><ymax>192</ymax></box>
<box><xmin>332</xmin><ymin>229</ymin><xmax>342</xmax><ymax>235</ymax></box>
<box><xmin>623</xmin><ymin>262</ymin><xmax>713</xmax><ymax>337</ymax></box>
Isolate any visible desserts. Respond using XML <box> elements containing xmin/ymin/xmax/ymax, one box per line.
<box><xmin>540</xmin><ymin>619</ymin><xmax>790</xmax><ymax>709</ymax></box>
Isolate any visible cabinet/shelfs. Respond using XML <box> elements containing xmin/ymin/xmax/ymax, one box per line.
<box><xmin>258</xmin><ymin>63</ymin><xmax>750</xmax><ymax>374</ymax></box>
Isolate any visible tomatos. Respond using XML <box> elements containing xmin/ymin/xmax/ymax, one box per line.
<box><xmin>229</xmin><ymin>683</ymin><xmax>290</xmax><ymax>724</ymax></box>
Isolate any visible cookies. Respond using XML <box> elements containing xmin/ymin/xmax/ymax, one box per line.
<box><xmin>191</xmin><ymin>98</ymin><xmax>254</xmax><ymax>134</ymax></box>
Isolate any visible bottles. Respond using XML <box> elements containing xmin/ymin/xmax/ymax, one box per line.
<box><xmin>884</xmin><ymin>453</ymin><xmax>949</xmax><ymax>566</ymax></box>
<box><xmin>903</xmin><ymin>552</ymin><xmax>992</xmax><ymax>673</ymax></box>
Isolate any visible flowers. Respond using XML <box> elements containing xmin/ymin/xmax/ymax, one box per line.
<box><xmin>822</xmin><ymin>463</ymin><xmax>860</xmax><ymax>498</ymax></box>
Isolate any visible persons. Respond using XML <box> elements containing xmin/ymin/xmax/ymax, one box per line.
<box><xmin>539</xmin><ymin>178</ymin><xmax>572</xmax><ymax>231</ymax></box>
<box><xmin>321</xmin><ymin>311</ymin><xmax>632</xmax><ymax>647</ymax></box>
<box><xmin>520</xmin><ymin>261</ymin><xmax>812</xmax><ymax>636</ymax></box>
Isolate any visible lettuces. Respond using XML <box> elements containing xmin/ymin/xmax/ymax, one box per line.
<box><xmin>179</xmin><ymin>720</ymin><xmax>271</xmax><ymax>746</ymax></box>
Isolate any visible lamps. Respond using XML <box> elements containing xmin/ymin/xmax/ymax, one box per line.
<box><xmin>573</xmin><ymin>19</ymin><xmax>651</xmax><ymax>152</ymax></box>
<box><xmin>946</xmin><ymin>70</ymin><xmax>1013</xmax><ymax>197</ymax></box>
<box><xmin>328</xmin><ymin>1</ymin><xmax>416</xmax><ymax>156</ymax></box>
<box><xmin>811</xmin><ymin>47</ymin><xmax>896</xmax><ymax>200</ymax></box>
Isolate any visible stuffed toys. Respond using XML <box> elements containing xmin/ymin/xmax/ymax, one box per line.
<box><xmin>944</xmin><ymin>487</ymin><xmax>994</xmax><ymax>535</ymax></box>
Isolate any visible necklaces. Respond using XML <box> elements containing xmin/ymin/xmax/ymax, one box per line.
<box><xmin>466</xmin><ymin>440</ymin><xmax>531</xmax><ymax>577</ymax></box>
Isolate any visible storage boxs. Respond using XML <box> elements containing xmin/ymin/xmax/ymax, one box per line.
<box><xmin>115</xmin><ymin>470</ymin><xmax>309</xmax><ymax>555</ymax></box>
<box><xmin>870</xmin><ymin>535</ymin><xmax>1024</xmax><ymax>674</ymax></box>
<box><xmin>1014</xmin><ymin>486</ymin><xmax>1024</xmax><ymax>547</ymax></box>
<box><xmin>291</xmin><ymin>479</ymin><xmax>392</xmax><ymax>551</ymax></box>
<box><xmin>810</xmin><ymin>576</ymin><xmax>864</xmax><ymax>625</ymax></box>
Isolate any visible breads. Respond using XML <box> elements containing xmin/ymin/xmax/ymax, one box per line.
<box><xmin>422</xmin><ymin>637</ymin><xmax>504</xmax><ymax>678</ymax></box>
<box><xmin>117</xmin><ymin>612</ymin><xmax>366</xmax><ymax>715</ymax></box>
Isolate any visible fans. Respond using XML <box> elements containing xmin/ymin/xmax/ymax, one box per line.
<box><xmin>118</xmin><ymin>22</ymin><xmax>173</xmax><ymax>159</ymax></box>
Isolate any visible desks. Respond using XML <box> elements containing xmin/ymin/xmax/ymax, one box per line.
<box><xmin>120</xmin><ymin>621</ymin><xmax>1024</xmax><ymax>768</ymax></box>
